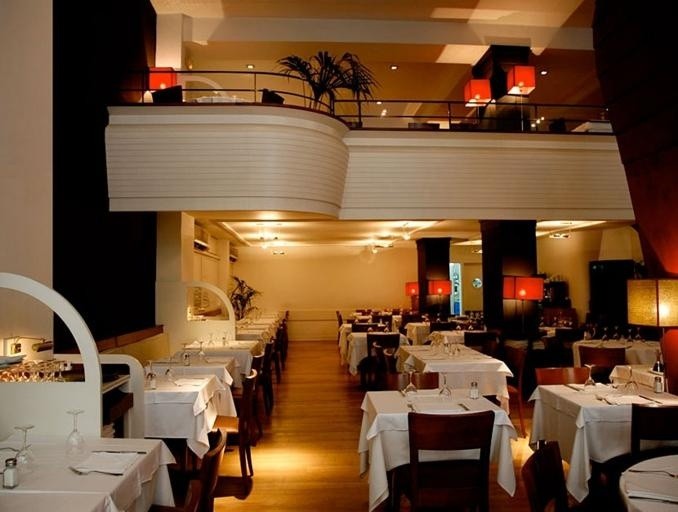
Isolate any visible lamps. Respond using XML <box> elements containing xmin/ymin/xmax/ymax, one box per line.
<box><xmin>148</xmin><ymin>67</ymin><xmax>177</xmax><ymax>93</ymax></box>
<box><xmin>463</xmin><ymin>65</ymin><xmax>536</xmax><ymax>108</ymax></box>
<box><xmin>626</xmin><ymin>278</ymin><xmax>678</xmax><ymax>328</ymax></box>
<box><xmin>405</xmin><ymin>280</ymin><xmax>452</xmax><ymax>296</ymax></box>
<box><xmin>502</xmin><ymin>275</ymin><xmax>544</xmax><ymax>329</ymax></box>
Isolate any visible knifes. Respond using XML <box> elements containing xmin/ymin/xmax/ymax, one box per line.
<box><xmin>92</xmin><ymin>450</ymin><xmax>147</xmax><ymax>454</ymax></box>
<box><xmin>628</xmin><ymin>495</ymin><xmax>678</xmax><ymax>506</ymax></box>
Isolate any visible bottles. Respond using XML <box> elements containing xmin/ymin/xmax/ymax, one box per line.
<box><xmin>469</xmin><ymin>382</ymin><xmax>479</xmax><ymax>398</ymax></box>
<box><xmin>653</xmin><ymin>350</ymin><xmax>664</xmax><ymax>372</ymax></box>
<box><xmin>653</xmin><ymin>377</ymin><xmax>663</xmax><ymax>394</ymax></box>
<box><xmin>2</xmin><ymin>458</ymin><xmax>20</xmax><ymax>489</ymax></box>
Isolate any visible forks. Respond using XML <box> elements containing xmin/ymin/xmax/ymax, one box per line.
<box><xmin>628</xmin><ymin>468</ymin><xmax>678</xmax><ymax>478</ymax></box>
<box><xmin>68</xmin><ymin>466</ymin><xmax>123</xmax><ymax>476</ymax></box>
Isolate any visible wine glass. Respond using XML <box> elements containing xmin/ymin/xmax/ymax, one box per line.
<box><xmin>600</xmin><ymin>325</ymin><xmax>643</xmax><ymax>348</ymax></box>
<box><xmin>182</xmin><ymin>340</ymin><xmax>206</xmax><ymax>366</ymax></box>
<box><xmin>13</xmin><ymin>426</ymin><xmax>36</xmax><ymax>476</ymax></box>
<box><xmin>0</xmin><ymin>359</ymin><xmax>67</xmax><ymax>383</ymax></box>
<box><xmin>583</xmin><ymin>364</ymin><xmax>597</xmax><ymax>394</ymax></box>
<box><xmin>65</xmin><ymin>409</ymin><xmax>88</xmax><ymax>461</ymax></box>
<box><xmin>437</xmin><ymin>369</ymin><xmax>453</xmax><ymax>403</ymax></box>
<box><xmin>624</xmin><ymin>365</ymin><xmax>639</xmax><ymax>397</ymax></box>
<box><xmin>207</xmin><ymin>331</ymin><xmax>230</xmax><ymax>352</ymax></box>
<box><xmin>403</xmin><ymin>369</ymin><xmax>419</xmax><ymax>402</ymax></box>
<box><xmin>145</xmin><ymin>355</ymin><xmax>177</xmax><ymax>390</ymax></box>
